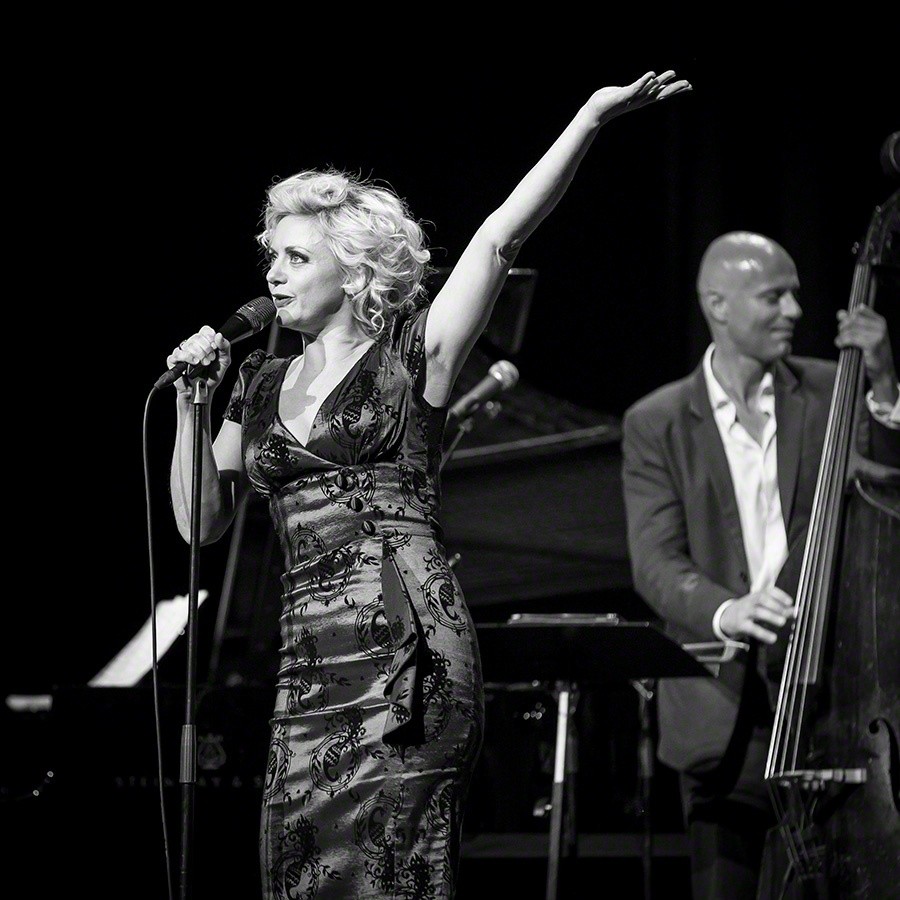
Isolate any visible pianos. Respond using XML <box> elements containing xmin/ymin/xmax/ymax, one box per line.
<box><xmin>474</xmin><ymin>613</ymin><xmax>715</xmax><ymax>677</ymax></box>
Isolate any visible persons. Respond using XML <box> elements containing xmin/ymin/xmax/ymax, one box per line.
<box><xmin>621</xmin><ymin>234</ymin><xmax>900</xmax><ymax>900</ymax></box>
<box><xmin>165</xmin><ymin>70</ymin><xmax>693</xmax><ymax>900</ymax></box>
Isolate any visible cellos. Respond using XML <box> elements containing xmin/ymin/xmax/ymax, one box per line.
<box><xmin>670</xmin><ymin>124</ymin><xmax>900</xmax><ymax>900</ymax></box>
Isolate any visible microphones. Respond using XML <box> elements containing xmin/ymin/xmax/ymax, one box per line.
<box><xmin>447</xmin><ymin>359</ymin><xmax>520</xmax><ymax>424</ymax></box>
<box><xmin>155</xmin><ymin>296</ymin><xmax>277</xmax><ymax>390</ymax></box>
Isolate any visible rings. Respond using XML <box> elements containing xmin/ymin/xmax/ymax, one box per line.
<box><xmin>179</xmin><ymin>340</ymin><xmax>187</xmax><ymax>350</ymax></box>
